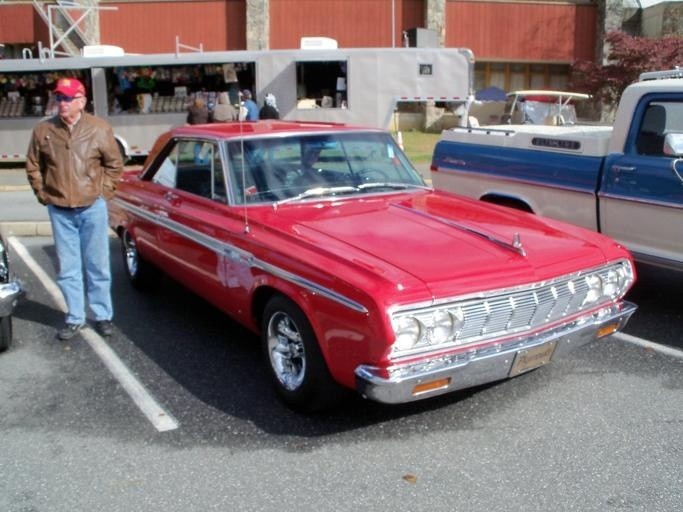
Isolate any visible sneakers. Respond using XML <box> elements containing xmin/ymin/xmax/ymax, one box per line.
<box><xmin>59</xmin><ymin>321</ymin><xmax>86</xmax><ymax>340</ymax></box>
<box><xmin>95</xmin><ymin>319</ymin><xmax>113</xmax><ymax>336</ymax></box>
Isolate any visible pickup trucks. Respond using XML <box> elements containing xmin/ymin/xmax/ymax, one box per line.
<box><xmin>424</xmin><ymin>66</ymin><xmax>682</xmax><ymax>296</ymax></box>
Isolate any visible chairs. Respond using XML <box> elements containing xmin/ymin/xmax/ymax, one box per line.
<box><xmin>635</xmin><ymin>105</ymin><xmax>669</xmax><ymax>157</ymax></box>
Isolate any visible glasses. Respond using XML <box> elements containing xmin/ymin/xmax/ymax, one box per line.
<box><xmin>54</xmin><ymin>94</ymin><xmax>82</xmax><ymax>102</ymax></box>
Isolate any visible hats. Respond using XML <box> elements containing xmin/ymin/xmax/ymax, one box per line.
<box><xmin>53</xmin><ymin>78</ymin><xmax>86</xmax><ymax>99</ymax></box>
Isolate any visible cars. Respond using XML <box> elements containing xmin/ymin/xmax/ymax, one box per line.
<box><xmin>104</xmin><ymin>115</ymin><xmax>641</xmax><ymax>420</ymax></box>
<box><xmin>0</xmin><ymin>231</ymin><xmax>23</xmax><ymax>353</ymax></box>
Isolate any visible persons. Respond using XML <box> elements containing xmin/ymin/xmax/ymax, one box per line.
<box><xmin>209</xmin><ymin>94</ymin><xmax>236</xmax><ymax>124</ymax></box>
<box><xmin>23</xmin><ymin>77</ymin><xmax>125</xmax><ymax>341</ymax></box>
<box><xmin>257</xmin><ymin>93</ymin><xmax>282</xmax><ymax>122</ymax></box>
<box><xmin>238</xmin><ymin>89</ymin><xmax>257</xmax><ymax>122</ymax></box>
<box><xmin>185</xmin><ymin>98</ymin><xmax>210</xmax><ymax>124</ymax></box>
